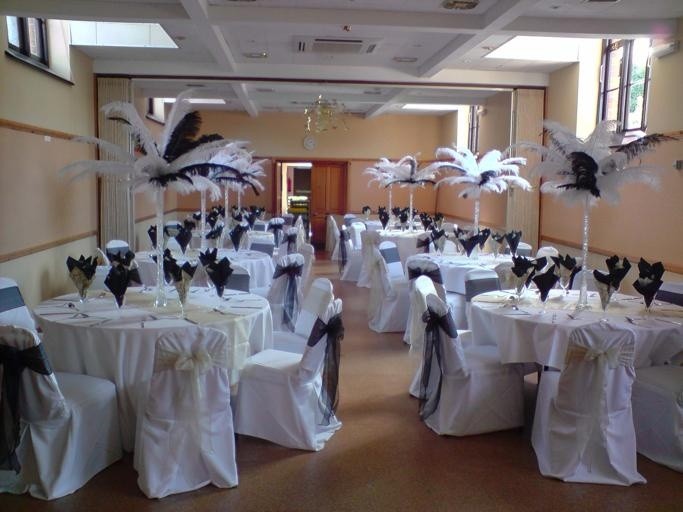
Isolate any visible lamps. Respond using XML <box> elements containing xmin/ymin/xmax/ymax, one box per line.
<box><xmin>303</xmin><ymin>94</ymin><xmax>349</xmax><ymax>135</ymax></box>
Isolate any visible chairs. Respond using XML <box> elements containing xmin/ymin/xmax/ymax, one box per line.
<box><xmin>655</xmin><ymin>281</ymin><xmax>682</xmax><ymax>307</ymax></box>
<box><xmin>68</xmin><ymin>203</ymin><xmax>314</xmax><ymax>332</ymax></box>
<box><xmin>324</xmin><ymin>205</ymin><xmax>560</xmax><ymax>352</ymax></box>
<box><xmin>417</xmin><ymin>293</ymin><xmax>526</xmax><ymax>437</ymax></box>
<box><xmin>232</xmin><ymin>290</ymin><xmax>345</xmax><ymax>452</ymax></box>
<box><xmin>570</xmin><ymin>267</ymin><xmax>609</xmax><ymax>292</ymax></box>
<box><xmin>410</xmin><ymin>274</ymin><xmax>472</xmax><ymax>400</ymax></box>
<box><xmin>0</xmin><ymin>277</ymin><xmax>43</xmax><ymax>337</ymax></box>
<box><xmin>273</xmin><ymin>276</ymin><xmax>335</xmax><ymax>353</ymax></box>
<box><xmin>530</xmin><ymin>318</ymin><xmax>649</xmax><ymax>486</ymax></box>
<box><xmin>1</xmin><ymin>324</ymin><xmax>128</xmax><ymax>501</ymax></box>
<box><xmin>132</xmin><ymin>325</ymin><xmax>240</xmax><ymax>499</ymax></box>
<box><xmin>631</xmin><ymin>363</ymin><xmax>682</xmax><ymax>473</ymax></box>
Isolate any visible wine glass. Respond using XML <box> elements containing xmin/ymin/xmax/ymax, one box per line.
<box><xmin>513</xmin><ymin>274</ymin><xmax>658</xmax><ymax>324</ymax></box>
<box><xmin>72</xmin><ymin>284</ymin><xmax>228</xmax><ymax>320</ymax></box>
